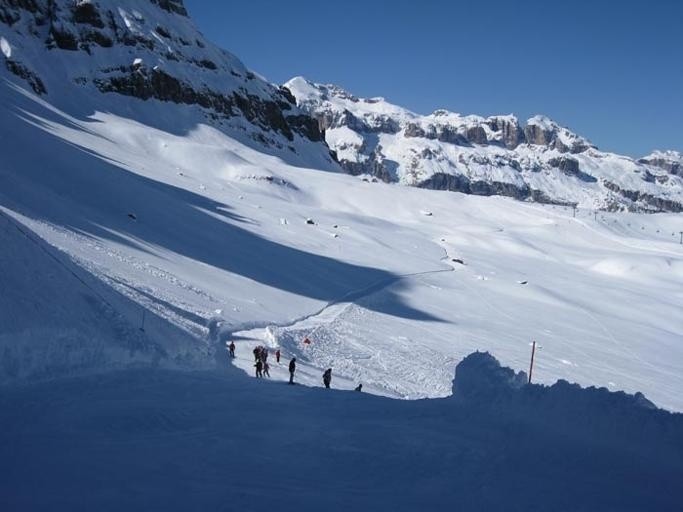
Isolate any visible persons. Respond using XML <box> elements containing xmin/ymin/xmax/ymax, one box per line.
<box><xmin>354</xmin><ymin>384</ymin><xmax>362</xmax><ymax>391</ymax></box>
<box><xmin>323</xmin><ymin>368</ymin><xmax>331</xmax><ymax>390</ymax></box>
<box><xmin>274</xmin><ymin>348</ymin><xmax>280</xmax><ymax>363</ymax></box>
<box><xmin>252</xmin><ymin>345</ymin><xmax>271</xmax><ymax>379</ymax></box>
<box><xmin>228</xmin><ymin>341</ymin><xmax>235</xmax><ymax>358</ymax></box>
<box><xmin>288</xmin><ymin>357</ymin><xmax>296</xmax><ymax>384</ymax></box>
